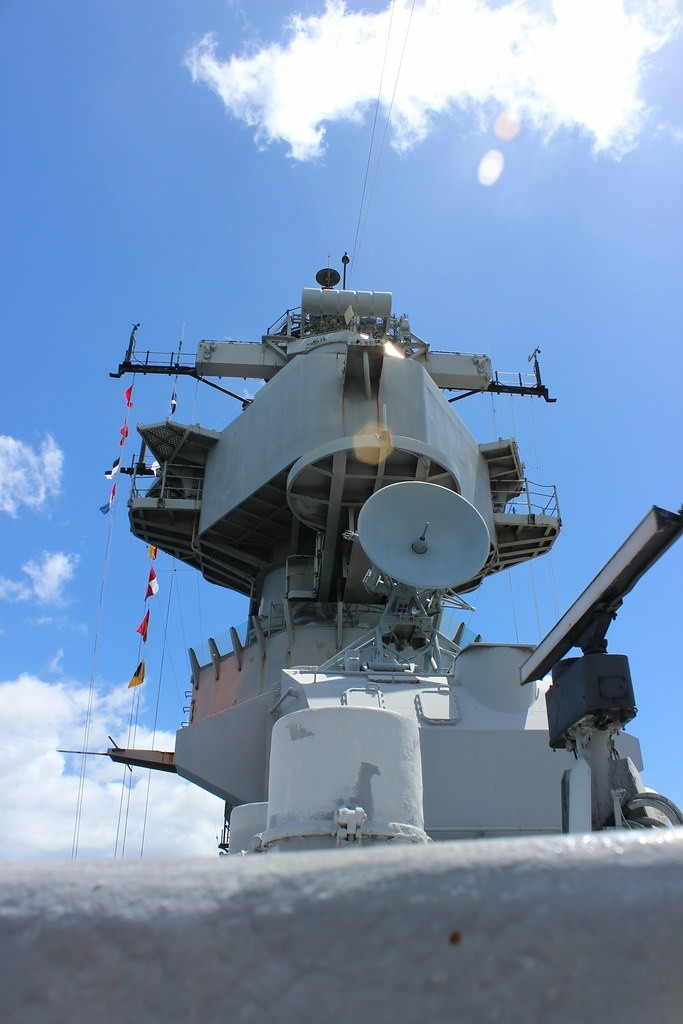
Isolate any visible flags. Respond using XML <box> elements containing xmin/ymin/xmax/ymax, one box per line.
<box><xmin>147</xmin><ymin>545</ymin><xmax>157</xmax><ymax>560</ymax></box>
<box><xmin>145</xmin><ymin>568</ymin><xmax>159</xmax><ymax>600</ymax></box>
<box><xmin>99</xmin><ymin>482</ymin><xmax>117</xmax><ymax>514</ymax></box>
<box><xmin>105</xmin><ymin>457</ymin><xmax>120</xmax><ymax>480</ymax></box>
<box><xmin>136</xmin><ymin>610</ymin><xmax>150</xmax><ymax>644</ymax></box>
<box><xmin>125</xmin><ymin>386</ymin><xmax>133</xmax><ymax>409</ymax></box>
<box><xmin>128</xmin><ymin>658</ymin><xmax>145</xmax><ymax>688</ymax></box>
<box><xmin>170</xmin><ymin>391</ymin><xmax>177</xmax><ymax>414</ymax></box>
<box><xmin>150</xmin><ymin>461</ymin><xmax>161</xmax><ymax>476</ymax></box>
<box><xmin>120</xmin><ymin>421</ymin><xmax>128</xmax><ymax>445</ymax></box>
